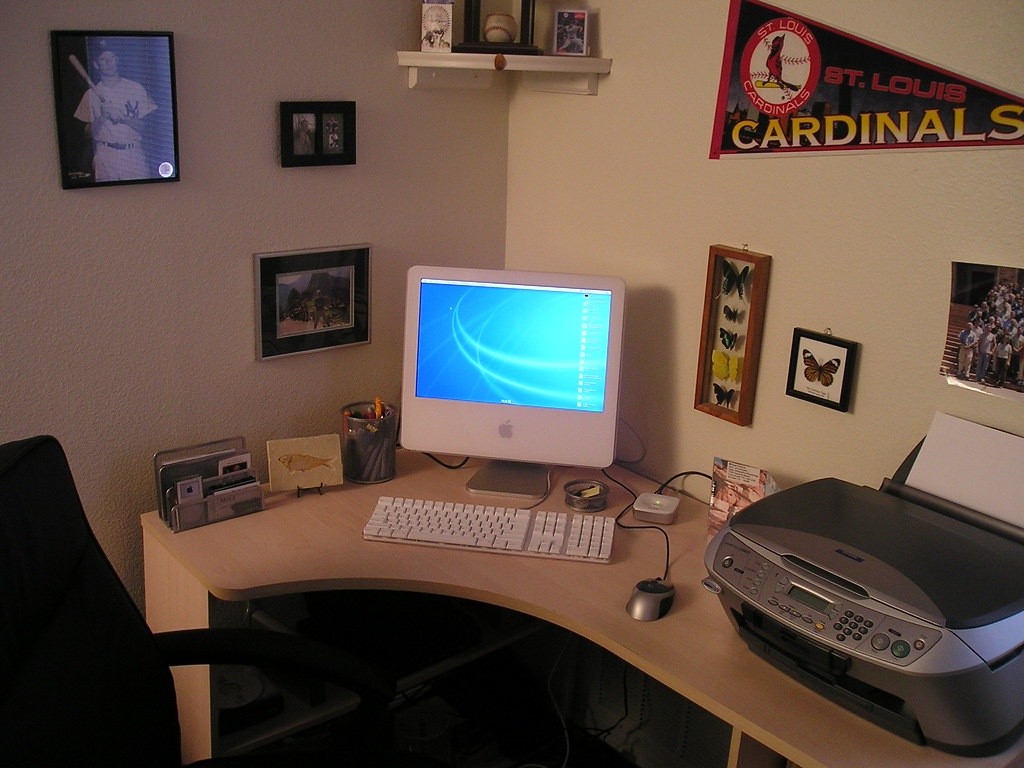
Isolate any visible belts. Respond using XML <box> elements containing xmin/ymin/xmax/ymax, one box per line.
<box><xmin>102</xmin><ymin>141</ymin><xmax>134</xmax><ymax>150</ymax></box>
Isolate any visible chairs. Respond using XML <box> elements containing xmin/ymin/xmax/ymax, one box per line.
<box><xmin>0</xmin><ymin>434</ymin><xmax>455</xmax><ymax>768</ymax></box>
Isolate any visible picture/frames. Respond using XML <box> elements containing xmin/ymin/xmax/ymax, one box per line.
<box><xmin>784</xmin><ymin>327</ymin><xmax>858</xmax><ymax>412</ymax></box>
<box><xmin>49</xmin><ymin>29</ymin><xmax>180</xmax><ymax>190</ymax></box>
<box><xmin>276</xmin><ymin>98</ymin><xmax>357</xmax><ymax>168</ymax></box>
<box><xmin>550</xmin><ymin>6</ymin><xmax>590</xmax><ymax>56</ymax></box>
<box><xmin>253</xmin><ymin>241</ymin><xmax>375</xmax><ymax>362</ymax></box>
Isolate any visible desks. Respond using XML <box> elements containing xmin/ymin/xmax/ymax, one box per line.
<box><xmin>138</xmin><ymin>451</ymin><xmax>1024</xmax><ymax>768</ymax></box>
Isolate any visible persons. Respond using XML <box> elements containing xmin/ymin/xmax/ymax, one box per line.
<box><xmin>312</xmin><ymin>289</ymin><xmax>330</xmax><ymax>329</ymax></box>
<box><xmin>73</xmin><ymin>40</ymin><xmax>158</xmax><ymax>182</ymax></box>
<box><xmin>294</xmin><ymin>116</ymin><xmax>339</xmax><ymax>157</ymax></box>
<box><xmin>957</xmin><ymin>282</ymin><xmax>1024</xmax><ymax>389</ymax></box>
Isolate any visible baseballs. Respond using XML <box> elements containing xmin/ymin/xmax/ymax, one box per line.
<box><xmin>482</xmin><ymin>12</ymin><xmax>519</xmax><ymax>43</ymax></box>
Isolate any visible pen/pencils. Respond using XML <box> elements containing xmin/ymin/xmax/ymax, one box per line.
<box><xmin>342</xmin><ymin>396</ymin><xmax>397</xmax><ymax>485</ymax></box>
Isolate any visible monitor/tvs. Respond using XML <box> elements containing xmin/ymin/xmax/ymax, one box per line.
<box><xmin>399</xmin><ymin>265</ymin><xmax>626</xmax><ymax>499</ymax></box>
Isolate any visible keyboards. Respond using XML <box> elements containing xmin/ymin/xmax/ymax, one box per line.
<box><xmin>361</xmin><ymin>497</ymin><xmax>615</xmax><ymax>564</ymax></box>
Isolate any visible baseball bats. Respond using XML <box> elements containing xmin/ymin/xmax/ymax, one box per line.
<box><xmin>69</xmin><ymin>54</ymin><xmax>121</xmax><ymax>125</ymax></box>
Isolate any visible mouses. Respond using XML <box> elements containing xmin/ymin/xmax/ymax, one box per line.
<box><xmin>625</xmin><ymin>577</ymin><xmax>675</xmax><ymax>621</ymax></box>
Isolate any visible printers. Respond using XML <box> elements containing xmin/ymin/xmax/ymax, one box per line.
<box><xmin>701</xmin><ymin>411</ymin><xmax>1024</xmax><ymax>758</ymax></box>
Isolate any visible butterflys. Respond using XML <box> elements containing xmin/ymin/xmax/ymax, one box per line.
<box><xmin>711</xmin><ymin>259</ymin><xmax>750</xmax><ymax>408</ymax></box>
<box><xmin>803</xmin><ymin>349</ymin><xmax>841</xmax><ymax>387</ymax></box>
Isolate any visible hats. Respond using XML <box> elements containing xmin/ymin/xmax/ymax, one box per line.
<box><xmin>92</xmin><ymin>37</ymin><xmax>121</xmax><ymax>57</ymax></box>
